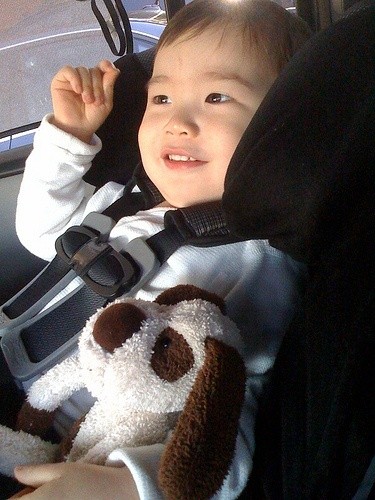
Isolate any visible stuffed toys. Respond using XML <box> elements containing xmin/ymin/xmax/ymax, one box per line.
<box><xmin>0</xmin><ymin>283</ymin><xmax>247</xmax><ymax>499</ymax></box>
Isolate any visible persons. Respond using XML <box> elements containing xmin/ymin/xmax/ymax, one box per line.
<box><xmin>0</xmin><ymin>0</ymin><xmax>315</xmax><ymax>500</ymax></box>
